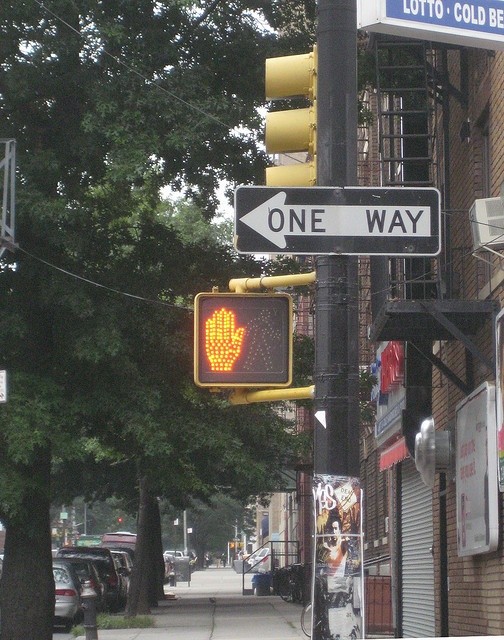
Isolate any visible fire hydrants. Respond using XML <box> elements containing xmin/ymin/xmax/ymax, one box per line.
<box><xmin>80</xmin><ymin>579</ymin><xmax>101</xmax><ymax>640</ymax></box>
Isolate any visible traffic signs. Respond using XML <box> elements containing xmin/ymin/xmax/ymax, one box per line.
<box><xmin>234</xmin><ymin>187</ymin><xmax>442</xmax><ymax>257</ymax></box>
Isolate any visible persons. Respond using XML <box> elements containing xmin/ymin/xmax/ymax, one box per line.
<box><xmin>320</xmin><ymin>517</ymin><xmax>352</xmax><ymax>592</ymax></box>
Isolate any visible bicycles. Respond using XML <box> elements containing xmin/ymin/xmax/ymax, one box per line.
<box><xmin>281</xmin><ymin>565</ymin><xmax>303</xmax><ymax>604</ymax></box>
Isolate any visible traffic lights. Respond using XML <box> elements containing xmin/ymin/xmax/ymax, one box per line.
<box><xmin>263</xmin><ymin>44</ymin><xmax>319</xmax><ymax>189</ymax></box>
<box><xmin>194</xmin><ymin>291</ymin><xmax>292</xmax><ymax>388</ymax></box>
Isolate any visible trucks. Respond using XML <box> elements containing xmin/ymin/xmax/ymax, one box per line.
<box><xmin>100</xmin><ymin>531</ymin><xmax>137</xmax><ymax>549</ymax></box>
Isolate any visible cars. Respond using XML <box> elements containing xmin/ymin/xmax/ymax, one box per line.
<box><xmin>53</xmin><ymin>556</ymin><xmax>105</xmax><ymax>611</ymax></box>
<box><xmin>255</xmin><ymin>548</ymin><xmax>274</xmax><ymax>574</ymax></box>
<box><xmin>164</xmin><ymin>551</ymin><xmax>190</xmax><ymax>562</ymax></box>
<box><xmin>109</xmin><ymin>549</ymin><xmax>133</xmax><ymax>608</ymax></box>
<box><xmin>57</xmin><ymin>546</ymin><xmax>122</xmax><ymax>613</ymax></box>
<box><xmin>50</xmin><ymin>562</ymin><xmax>80</xmax><ymax>634</ymax></box>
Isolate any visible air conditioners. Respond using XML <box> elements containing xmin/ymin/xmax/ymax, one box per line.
<box><xmin>468</xmin><ymin>196</ymin><xmax>503</xmax><ymax>252</ymax></box>
